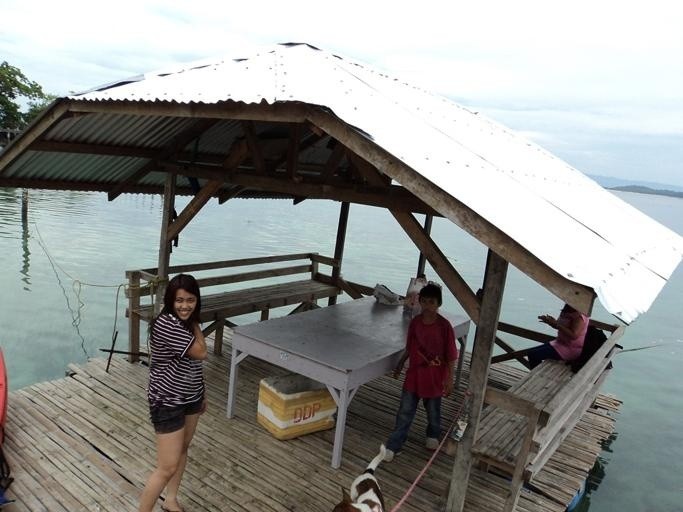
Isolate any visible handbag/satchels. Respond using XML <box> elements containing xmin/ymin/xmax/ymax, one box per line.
<box><xmin>577</xmin><ymin>325</ymin><xmax>613</xmax><ymax>369</ymax></box>
<box><xmin>373</xmin><ymin>283</ymin><xmax>400</xmax><ymax>306</ymax></box>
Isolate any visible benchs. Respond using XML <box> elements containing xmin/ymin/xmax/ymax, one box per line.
<box><xmin>444</xmin><ymin>317</ymin><xmax>626</xmax><ymax>511</ymax></box>
<box><xmin>124</xmin><ymin>253</ymin><xmax>343</xmax><ymax>364</ymax></box>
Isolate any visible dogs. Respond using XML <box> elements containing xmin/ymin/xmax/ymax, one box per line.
<box><xmin>331</xmin><ymin>442</ymin><xmax>387</xmax><ymax>512</ymax></box>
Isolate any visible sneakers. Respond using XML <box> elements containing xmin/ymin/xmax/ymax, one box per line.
<box><xmin>383</xmin><ymin>447</ymin><xmax>404</xmax><ymax>463</ymax></box>
<box><xmin>425</xmin><ymin>435</ymin><xmax>440</xmax><ymax>450</ymax></box>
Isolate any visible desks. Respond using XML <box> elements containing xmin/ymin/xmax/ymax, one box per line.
<box><xmin>226</xmin><ymin>295</ymin><xmax>470</xmax><ymax>468</ymax></box>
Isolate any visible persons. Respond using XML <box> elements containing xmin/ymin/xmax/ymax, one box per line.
<box><xmin>0</xmin><ymin>349</ymin><xmax>8</xmax><ymax>508</ymax></box>
<box><xmin>383</xmin><ymin>286</ymin><xmax>456</xmax><ymax>462</ymax></box>
<box><xmin>140</xmin><ymin>275</ymin><xmax>208</xmax><ymax>512</ymax></box>
<box><xmin>529</xmin><ymin>305</ymin><xmax>590</xmax><ymax>370</ymax></box>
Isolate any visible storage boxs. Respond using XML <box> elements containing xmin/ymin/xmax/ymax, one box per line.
<box><xmin>258</xmin><ymin>371</ymin><xmax>338</xmax><ymax>441</ymax></box>
